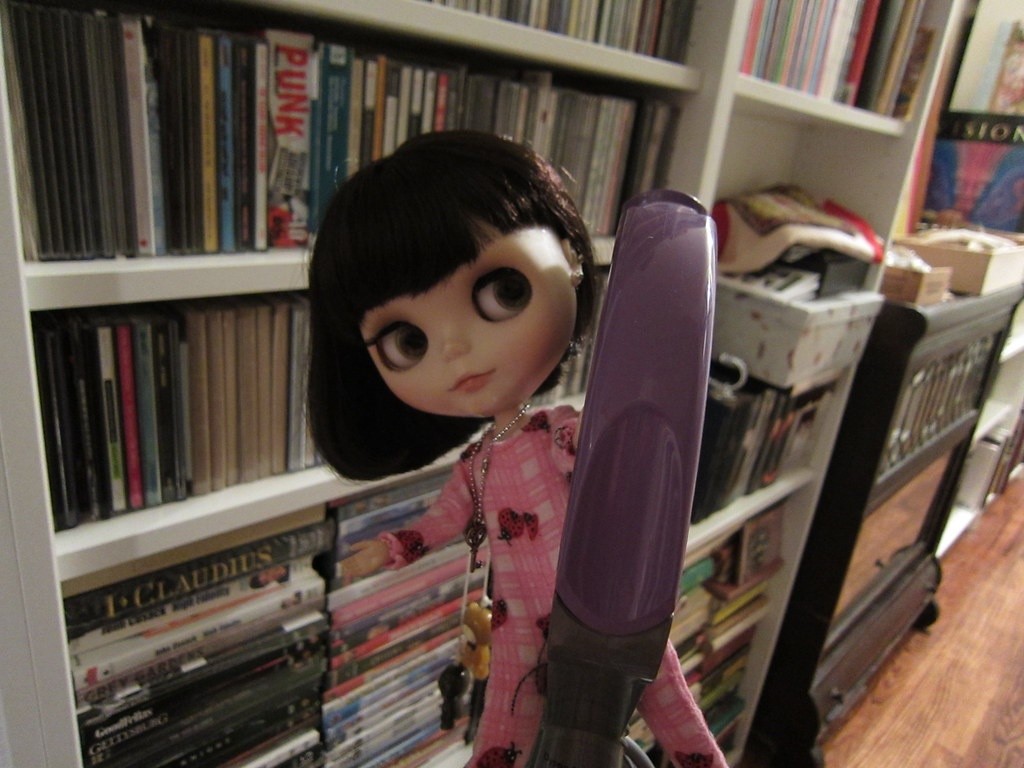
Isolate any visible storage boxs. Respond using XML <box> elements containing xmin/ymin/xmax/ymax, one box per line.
<box><xmin>889</xmin><ymin>224</ymin><xmax>1024</xmax><ymax>295</ymax></box>
<box><xmin>711</xmin><ymin>276</ymin><xmax>883</xmax><ymax>395</ymax></box>
<box><xmin>880</xmin><ymin>264</ymin><xmax>951</xmax><ymax>307</ymax></box>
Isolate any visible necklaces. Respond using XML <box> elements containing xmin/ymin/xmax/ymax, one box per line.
<box><xmin>467</xmin><ymin>405</ymin><xmax>529</xmax><ymax>574</ymax></box>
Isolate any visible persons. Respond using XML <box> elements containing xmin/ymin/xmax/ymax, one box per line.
<box><xmin>306</xmin><ymin>130</ymin><xmax>732</xmax><ymax>768</ymax></box>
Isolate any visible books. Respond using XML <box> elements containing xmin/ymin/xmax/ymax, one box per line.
<box><xmin>624</xmin><ymin>501</ymin><xmax>786</xmax><ymax>767</ymax></box>
<box><xmin>685</xmin><ymin>374</ymin><xmax>828</xmax><ymax>527</ymax></box>
<box><xmin>30</xmin><ymin>296</ymin><xmax>326</xmax><ymax>531</ymax></box>
<box><xmin>739</xmin><ymin>1</ymin><xmax>931</xmax><ymax>122</ymax></box>
<box><xmin>424</xmin><ymin>0</ymin><xmax>694</xmax><ymax>66</ymax></box>
<box><xmin>422</xmin><ymin>72</ymin><xmax>677</xmax><ymax>236</ymax></box>
<box><xmin>59</xmin><ymin>503</ymin><xmax>334</xmax><ymax>768</ymax></box>
<box><xmin>0</xmin><ymin>1</ymin><xmax>420</xmax><ymax>263</ymax></box>
<box><xmin>320</xmin><ymin>472</ymin><xmax>489</xmax><ymax>768</ymax></box>
<box><xmin>525</xmin><ymin>269</ymin><xmax>608</xmax><ymax>409</ymax></box>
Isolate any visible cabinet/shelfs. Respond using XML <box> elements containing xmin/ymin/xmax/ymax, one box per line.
<box><xmin>0</xmin><ymin>0</ymin><xmax>1024</xmax><ymax>768</ymax></box>
